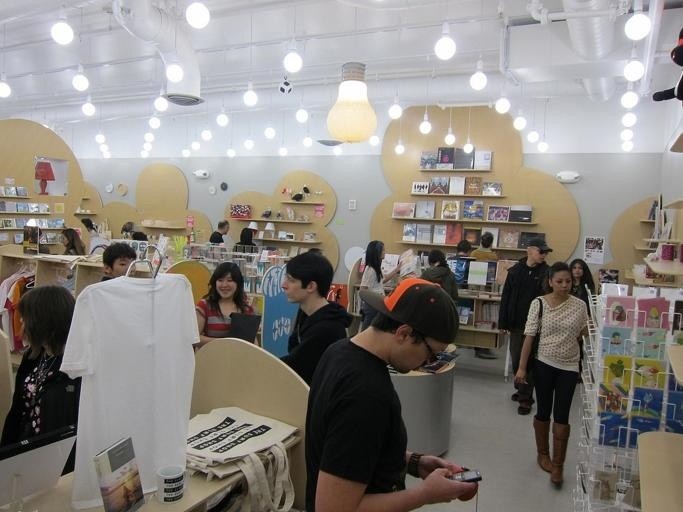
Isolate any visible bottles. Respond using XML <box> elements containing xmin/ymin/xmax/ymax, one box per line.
<box><xmin>190</xmin><ymin>227</ymin><xmax>195</xmax><ymax>243</ymax></box>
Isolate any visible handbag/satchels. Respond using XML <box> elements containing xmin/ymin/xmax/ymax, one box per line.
<box><xmin>517</xmin><ymin>332</ymin><xmax>539</xmax><ymax>372</ymax></box>
<box><xmin>187</xmin><ymin>406</ymin><xmax>298</xmax><ymax>512</ymax></box>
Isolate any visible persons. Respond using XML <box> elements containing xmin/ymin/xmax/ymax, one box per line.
<box><xmin>568</xmin><ymin>259</ymin><xmax>594</xmax><ymax>384</ymax></box>
<box><xmin>0</xmin><ymin>286</ymin><xmax>75</xmax><ymax>462</ymax></box>
<box><xmin>419</xmin><ymin>249</ymin><xmax>459</xmax><ymax>302</ymax></box>
<box><xmin>233</xmin><ymin>227</ymin><xmax>258</xmax><ymax>253</ymax></box>
<box><xmin>133</xmin><ymin>233</ymin><xmax>146</xmax><ymax>241</ymax></box>
<box><xmin>470</xmin><ymin>233</ymin><xmax>500</xmax><ymax>262</ymax></box>
<box><xmin>514</xmin><ymin>262</ymin><xmax>589</xmax><ymax>485</ymax></box>
<box><xmin>81</xmin><ymin>219</ymin><xmax>97</xmax><ymax>233</ymax></box>
<box><xmin>305</xmin><ymin>278</ymin><xmax>477</xmax><ymax>511</ymax></box>
<box><xmin>280</xmin><ymin>251</ymin><xmax>353</xmax><ymax>385</ymax></box>
<box><xmin>192</xmin><ymin>262</ymin><xmax>258</xmax><ymax>349</ymax></box>
<box><xmin>101</xmin><ymin>242</ymin><xmax>135</xmax><ymax>281</ymax></box>
<box><xmin>358</xmin><ymin>240</ymin><xmax>403</xmax><ymax>331</ymax></box>
<box><xmin>498</xmin><ymin>238</ymin><xmax>553</xmax><ymax>416</ymax></box>
<box><xmin>447</xmin><ymin>240</ymin><xmax>471</xmax><ymax>260</ymax></box>
<box><xmin>62</xmin><ymin>228</ymin><xmax>85</xmax><ymax>255</ymax></box>
<box><xmin>209</xmin><ymin>220</ymin><xmax>229</xmax><ymax>243</ymax></box>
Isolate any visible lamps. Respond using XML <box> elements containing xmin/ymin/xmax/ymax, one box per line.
<box><xmin>327</xmin><ymin>62</ymin><xmax>376</xmax><ymax>143</ymax></box>
<box><xmin>186</xmin><ymin>2</ymin><xmax>210</xmax><ymax>29</ymax></box>
<box><xmin>283</xmin><ymin>38</ymin><xmax>302</xmax><ymax>73</ymax></box>
<box><xmin>435</xmin><ymin>21</ymin><xmax>456</xmax><ymax>61</ymax></box>
<box><xmin>51</xmin><ymin>7</ymin><xmax>74</xmax><ymax>44</ymax></box>
<box><xmin>469</xmin><ymin>59</ymin><xmax>486</xmax><ymax>89</ymax></box>
<box><xmin>244</xmin><ymin>80</ymin><xmax>257</xmax><ymax>105</ymax></box>
<box><xmin>35</xmin><ymin>162</ymin><xmax>55</xmax><ymax>194</ymax></box>
<box><xmin>0</xmin><ymin>73</ymin><xmax>10</xmax><ymax>97</ymax></box>
<box><xmin>165</xmin><ymin>63</ymin><xmax>184</xmax><ymax>83</ymax></box>
<box><xmin>154</xmin><ymin>89</ymin><xmax>168</xmax><ymax>111</ymax></box>
<box><xmin>72</xmin><ymin>64</ymin><xmax>89</xmax><ymax>92</ymax></box>
<box><xmin>621</xmin><ymin>0</ymin><xmax>651</xmax><ymax>108</ymax></box>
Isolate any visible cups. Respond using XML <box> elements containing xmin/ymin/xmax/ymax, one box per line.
<box><xmin>659</xmin><ymin>243</ymin><xmax>676</xmax><ymax>262</ymax></box>
<box><xmin>679</xmin><ymin>243</ymin><xmax>682</xmax><ymax>262</ymax></box>
<box><xmin>156</xmin><ymin>464</ymin><xmax>185</xmax><ymax>504</ymax></box>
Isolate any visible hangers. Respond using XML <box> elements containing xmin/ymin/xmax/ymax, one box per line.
<box><xmin>18</xmin><ymin>262</ymin><xmax>35</xmax><ymax>288</ymax></box>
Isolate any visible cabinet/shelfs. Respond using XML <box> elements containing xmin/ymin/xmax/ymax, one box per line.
<box><xmin>345</xmin><ymin>258</ymin><xmax>511</xmax><ymax>384</ymax></box>
<box><xmin>0</xmin><ymin>244</ymin><xmax>150</xmax><ymax>300</ymax></box>
<box><xmin>232</xmin><ymin>202</ymin><xmax>324</xmax><ymax>244</ymax></box>
<box><xmin>574</xmin><ymin>284</ymin><xmax>682</xmax><ymax>512</ymax></box>
<box><xmin>75</xmin><ymin>197</ymin><xmax>96</xmax><ymax>215</ymax></box>
<box><xmin>0</xmin><ymin>197</ymin><xmax>64</xmax><ymax>244</ymax></box>
<box><xmin>625</xmin><ymin>220</ymin><xmax>680</xmax><ymax>288</ymax></box>
<box><xmin>393</xmin><ymin>169</ymin><xmax>537</xmax><ymax>252</ymax></box>
<box><xmin>0</xmin><ymin>337</ymin><xmax>311</xmax><ymax>512</ymax></box>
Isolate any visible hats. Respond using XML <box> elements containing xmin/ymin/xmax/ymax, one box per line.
<box><xmin>527</xmin><ymin>238</ymin><xmax>553</xmax><ymax>252</ymax></box>
<box><xmin>360</xmin><ymin>279</ymin><xmax>458</xmax><ymax>342</ymax></box>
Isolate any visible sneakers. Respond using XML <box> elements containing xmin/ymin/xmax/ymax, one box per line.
<box><xmin>512</xmin><ymin>391</ymin><xmax>519</xmax><ymax>401</ymax></box>
<box><xmin>518</xmin><ymin>401</ymin><xmax>530</xmax><ymax>414</ymax></box>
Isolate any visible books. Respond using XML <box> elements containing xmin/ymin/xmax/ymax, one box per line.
<box><xmin>443</xmin><ymin>258</ymin><xmax>519</xmax><ymax>328</ymax></box>
<box><xmin>0</xmin><ymin>176</ymin><xmax>66</xmax><ymax>254</ymax></box>
<box><xmin>93</xmin><ymin>435</ymin><xmax>144</xmax><ymax>512</ymax></box>
<box><xmin>391</xmin><ymin>144</ymin><xmax>533</xmax><ymax>248</ymax></box>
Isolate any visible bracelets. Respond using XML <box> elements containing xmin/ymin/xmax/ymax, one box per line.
<box><xmin>407</xmin><ymin>453</ymin><xmax>424</xmax><ymax>478</ymax></box>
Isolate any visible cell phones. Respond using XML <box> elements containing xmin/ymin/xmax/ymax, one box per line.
<box><xmin>446</xmin><ymin>469</ymin><xmax>483</xmax><ymax>483</ymax></box>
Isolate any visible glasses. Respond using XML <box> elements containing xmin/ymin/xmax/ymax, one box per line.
<box><xmin>535</xmin><ymin>248</ymin><xmax>545</xmax><ymax>254</ymax></box>
<box><xmin>420</xmin><ymin>335</ymin><xmax>439</xmax><ymax>365</ymax></box>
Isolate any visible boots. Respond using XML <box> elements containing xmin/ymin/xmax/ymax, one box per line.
<box><xmin>533</xmin><ymin>416</ymin><xmax>552</xmax><ymax>472</ymax></box>
<box><xmin>552</xmin><ymin>423</ymin><xmax>570</xmax><ymax>485</ymax></box>
